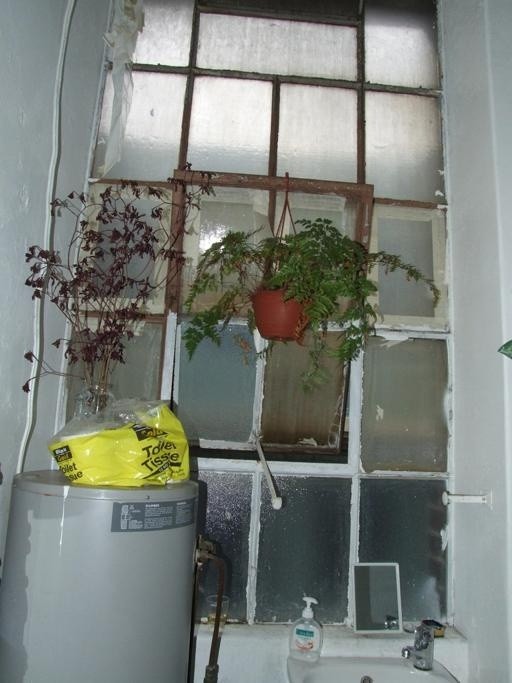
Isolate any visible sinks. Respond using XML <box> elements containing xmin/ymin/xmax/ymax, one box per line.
<box><xmin>285</xmin><ymin>655</ymin><xmax>462</xmax><ymax>683</ymax></box>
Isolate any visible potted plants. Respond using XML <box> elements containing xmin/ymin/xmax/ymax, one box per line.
<box><xmin>182</xmin><ymin>171</ymin><xmax>441</xmax><ymax>393</ymax></box>
<box><xmin>17</xmin><ymin>163</ymin><xmax>217</xmax><ymax>420</ymax></box>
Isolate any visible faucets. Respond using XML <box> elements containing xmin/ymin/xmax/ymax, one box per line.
<box><xmin>398</xmin><ymin>618</ymin><xmax>445</xmax><ymax>672</ymax></box>
<box><xmin>382</xmin><ymin>614</ymin><xmax>398</xmax><ymax>629</ymax></box>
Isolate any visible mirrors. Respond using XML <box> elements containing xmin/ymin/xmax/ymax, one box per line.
<box><xmin>349</xmin><ymin>561</ymin><xmax>403</xmax><ymax>636</ymax></box>
